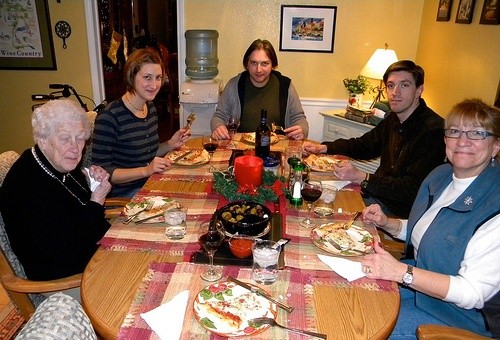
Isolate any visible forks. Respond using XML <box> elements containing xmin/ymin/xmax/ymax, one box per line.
<box><xmin>185</xmin><ymin>113</ymin><xmax>195</xmax><ymax>131</ymax></box>
<box><xmin>275</xmin><ymin>126</ymin><xmax>284</xmax><ymax>132</ymax></box>
<box><xmin>124</xmin><ymin>201</ymin><xmax>155</xmax><ymax>225</ymax></box>
<box><xmin>255</xmin><ymin>317</ymin><xmax>327</xmax><ymax>340</ymax></box>
<box><xmin>328</xmin><ymin>237</ymin><xmax>369</xmax><ymax>255</ymax></box>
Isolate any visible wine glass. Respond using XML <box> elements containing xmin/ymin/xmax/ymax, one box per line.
<box><xmin>225</xmin><ymin>114</ymin><xmax>240</xmax><ymax>147</ymax></box>
<box><xmin>300</xmin><ymin>178</ymin><xmax>323</xmax><ymax>228</ymax></box>
<box><xmin>198</xmin><ymin>220</ymin><xmax>224</xmax><ymax>282</ymax></box>
<box><xmin>202</xmin><ymin>134</ymin><xmax>219</xmax><ymax>168</ymax></box>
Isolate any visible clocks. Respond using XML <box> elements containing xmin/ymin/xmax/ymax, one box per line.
<box><xmin>54</xmin><ymin>20</ymin><xmax>72</xmax><ymax>49</ymax></box>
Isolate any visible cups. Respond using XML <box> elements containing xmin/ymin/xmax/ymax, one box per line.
<box><xmin>251</xmin><ymin>240</ymin><xmax>282</xmax><ymax>285</ymax></box>
<box><xmin>163</xmin><ymin>207</ymin><xmax>187</xmax><ymax>239</ymax></box>
<box><xmin>313</xmin><ymin>184</ymin><xmax>336</xmax><ymax>218</ymax></box>
<box><xmin>284</xmin><ymin>146</ymin><xmax>302</xmax><ymax>179</ymax></box>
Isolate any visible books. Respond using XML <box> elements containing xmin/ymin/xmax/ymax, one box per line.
<box><xmin>344</xmin><ymin>105</ymin><xmax>374</xmax><ymax>124</ymax></box>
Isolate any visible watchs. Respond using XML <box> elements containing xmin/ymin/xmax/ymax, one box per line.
<box><xmin>401</xmin><ymin>263</ymin><xmax>414</xmax><ymax>286</ymax></box>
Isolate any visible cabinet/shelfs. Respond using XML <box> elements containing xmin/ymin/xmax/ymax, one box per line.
<box><xmin>380</xmin><ymin>232</ymin><xmax>500</xmax><ymax>340</ymax></box>
<box><xmin>319</xmin><ymin>109</ymin><xmax>381</xmax><ymax>175</ymax></box>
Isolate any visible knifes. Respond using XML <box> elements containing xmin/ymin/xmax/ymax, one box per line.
<box><xmin>135</xmin><ymin>207</ymin><xmax>184</xmax><ymax>224</ymax></box>
<box><xmin>174</xmin><ymin>151</ymin><xmax>193</xmax><ymax>163</ymax></box>
<box><xmin>344</xmin><ymin>212</ymin><xmax>362</xmax><ymax>229</ymax></box>
<box><xmin>227</xmin><ymin>276</ymin><xmax>294</xmax><ymax>312</ymax></box>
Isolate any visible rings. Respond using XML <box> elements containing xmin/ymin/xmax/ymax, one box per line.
<box><xmin>295</xmin><ymin>135</ymin><xmax>299</xmax><ymax>138</ymax></box>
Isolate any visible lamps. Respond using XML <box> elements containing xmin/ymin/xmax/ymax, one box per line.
<box><xmin>358</xmin><ymin>47</ymin><xmax>400</xmax><ymax>108</ymax></box>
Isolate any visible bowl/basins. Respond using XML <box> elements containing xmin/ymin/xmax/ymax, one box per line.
<box><xmin>218</xmin><ymin>200</ymin><xmax>271</xmax><ymax>235</ymax></box>
<box><xmin>229</xmin><ymin>235</ymin><xmax>255</xmax><ymax>258</ymax></box>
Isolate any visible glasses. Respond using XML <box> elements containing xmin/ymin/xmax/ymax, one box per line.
<box><xmin>444</xmin><ymin>128</ymin><xmax>500</xmax><ymax>140</ymax></box>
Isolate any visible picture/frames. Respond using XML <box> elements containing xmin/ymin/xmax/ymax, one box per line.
<box><xmin>479</xmin><ymin>0</ymin><xmax>500</xmax><ymax>25</ymax></box>
<box><xmin>435</xmin><ymin>0</ymin><xmax>452</xmax><ymax>22</ymax></box>
<box><xmin>454</xmin><ymin>0</ymin><xmax>476</xmax><ymax>24</ymax></box>
<box><xmin>0</xmin><ymin>0</ymin><xmax>57</xmax><ymax>70</ymax></box>
<box><xmin>279</xmin><ymin>3</ymin><xmax>337</xmax><ymax>54</ymax></box>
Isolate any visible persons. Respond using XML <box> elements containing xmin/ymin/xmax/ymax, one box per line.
<box><xmin>302</xmin><ymin>60</ymin><xmax>453</xmax><ymax>220</ymax></box>
<box><xmin>91</xmin><ymin>47</ymin><xmax>190</xmax><ymax>221</ymax></box>
<box><xmin>210</xmin><ymin>39</ymin><xmax>309</xmax><ymax>140</ymax></box>
<box><xmin>362</xmin><ymin>97</ymin><xmax>500</xmax><ymax>339</ymax></box>
<box><xmin>0</xmin><ymin>97</ymin><xmax>113</xmax><ymax>304</ymax></box>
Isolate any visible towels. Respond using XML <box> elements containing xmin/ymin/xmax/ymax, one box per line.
<box><xmin>140</xmin><ymin>289</ymin><xmax>190</xmax><ymax>340</ymax></box>
<box><xmin>307</xmin><ymin>179</ymin><xmax>352</xmax><ymax>192</ymax></box>
<box><xmin>315</xmin><ymin>253</ymin><xmax>370</xmax><ymax>283</ymax></box>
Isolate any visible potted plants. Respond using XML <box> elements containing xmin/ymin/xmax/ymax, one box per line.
<box><xmin>343</xmin><ymin>74</ymin><xmax>371</xmax><ymax>109</ymax></box>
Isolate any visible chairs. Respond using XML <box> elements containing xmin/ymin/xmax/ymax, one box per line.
<box><xmin>13</xmin><ymin>291</ymin><xmax>98</xmax><ymax>340</ymax></box>
<box><xmin>79</xmin><ymin>111</ymin><xmax>131</xmax><ymax>207</ymax></box>
<box><xmin>0</xmin><ymin>147</ymin><xmax>125</xmax><ymax>324</ymax></box>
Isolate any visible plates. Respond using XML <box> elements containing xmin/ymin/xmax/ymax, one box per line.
<box><xmin>310</xmin><ymin>222</ymin><xmax>374</xmax><ymax>257</ymax></box>
<box><xmin>123</xmin><ymin>196</ymin><xmax>179</xmax><ymax>223</ymax></box>
<box><xmin>242</xmin><ymin>132</ymin><xmax>277</xmax><ymax>143</ymax></box>
<box><xmin>167</xmin><ymin>149</ymin><xmax>208</xmax><ymax>165</ymax></box>
<box><xmin>224</xmin><ymin>222</ymin><xmax>272</xmax><ymax>241</ymax></box>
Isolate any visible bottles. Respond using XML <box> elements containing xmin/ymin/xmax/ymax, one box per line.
<box><xmin>285</xmin><ymin>154</ymin><xmax>300</xmax><ymax>199</ymax></box>
<box><xmin>255</xmin><ymin>109</ymin><xmax>271</xmax><ymax>158</ymax></box>
<box><xmin>290</xmin><ymin>164</ymin><xmax>303</xmax><ymax>205</ymax></box>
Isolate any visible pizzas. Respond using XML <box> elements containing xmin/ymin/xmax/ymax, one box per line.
<box><xmin>241</xmin><ymin>132</ymin><xmax>279</xmax><ymax>144</ymax></box>
<box><xmin>164</xmin><ymin>149</ymin><xmax>211</xmax><ymax>165</ymax></box>
<box><xmin>124</xmin><ymin>201</ymin><xmax>181</xmax><ymax>223</ymax></box>
<box><xmin>304</xmin><ymin>153</ymin><xmax>349</xmax><ymax>172</ymax></box>
<box><xmin>313</xmin><ymin>222</ymin><xmax>354</xmax><ymax>251</ymax></box>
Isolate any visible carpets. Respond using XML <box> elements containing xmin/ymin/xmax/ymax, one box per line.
<box><xmin>0</xmin><ymin>285</ymin><xmax>26</xmax><ymax>340</ymax></box>
